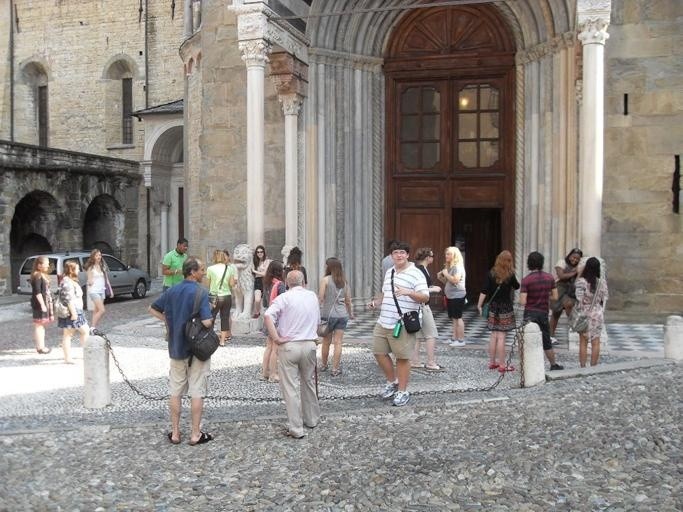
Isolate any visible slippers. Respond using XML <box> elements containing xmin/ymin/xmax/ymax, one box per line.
<box><xmin>189</xmin><ymin>430</ymin><xmax>213</xmax><ymax>446</ymax></box>
<box><xmin>167</xmin><ymin>431</ymin><xmax>181</xmax><ymax>444</ymax></box>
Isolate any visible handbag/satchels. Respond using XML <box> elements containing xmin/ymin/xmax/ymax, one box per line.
<box><xmin>404</xmin><ymin>311</ymin><xmax>421</xmax><ymax>333</ymax></box>
<box><xmin>569</xmin><ymin>306</ymin><xmax>589</xmax><ymax>333</ymax></box>
<box><xmin>56</xmin><ymin>302</ymin><xmax>71</xmax><ymax>319</ymax></box>
<box><xmin>317</xmin><ymin>319</ymin><xmax>329</xmax><ymax>337</ymax></box>
<box><xmin>208</xmin><ymin>294</ymin><xmax>219</xmax><ymax>311</ymax></box>
<box><xmin>481</xmin><ymin>303</ymin><xmax>491</xmax><ymax>319</ymax></box>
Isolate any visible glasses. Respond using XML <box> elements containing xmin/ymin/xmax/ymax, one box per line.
<box><xmin>428</xmin><ymin>254</ymin><xmax>434</xmax><ymax>258</ymax></box>
<box><xmin>256</xmin><ymin>251</ymin><xmax>264</xmax><ymax>254</ymax></box>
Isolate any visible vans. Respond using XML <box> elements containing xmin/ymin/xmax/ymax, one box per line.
<box><xmin>17</xmin><ymin>252</ymin><xmax>152</xmax><ymax>301</ymax></box>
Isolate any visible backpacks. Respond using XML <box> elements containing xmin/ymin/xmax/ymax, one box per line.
<box><xmin>184</xmin><ymin>313</ymin><xmax>220</xmax><ymax>362</ymax></box>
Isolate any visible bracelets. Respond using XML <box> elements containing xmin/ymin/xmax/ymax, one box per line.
<box><xmin>174</xmin><ymin>270</ymin><xmax>177</xmax><ymax>276</ymax></box>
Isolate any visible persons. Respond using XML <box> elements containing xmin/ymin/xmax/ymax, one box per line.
<box><xmin>316</xmin><ymin>254</ymin><xmax>354</xmax><ymax>376</ymax></box>
<box><xmin>257</xmin><ymin>259</ymin><xmax>287</xmax><ymax>383</ymax></box>
<box><xmin>435</xmin><ymin>246</ymin><xmax>467</xmax><ymax>344</ymax></box>
<box><xmin>203</xmin><ymin>249</ymin><xmax>234</xmax><ymax>348</ymax></box>
<box><xmin>220</xmin><ymin>250</ymin><xmax>239</xmax><ymax>342</ymax></box>
<box><xmin>409</xmin><ymin>246</ymin><xmax>446</xmax><ymax>372</ymax></box>
<box><xmin>517</xmin><ymin>251</ymin><xmax>565</xmax><ymax>372</ymax></box>
<box><xmin>147</xmin><ymin>255</ymin><xmax>215</xmax><ymax>447</ymax></box>
<box><xmin>261</xmin><ymin>269</ymin><xmax>322</xmax><ymax>441</ymax></box>
<box><xmin>566</xmin><ymin>256</ymin><xmax>610</xmax><ymax>370</ymax></box>
<box><xmin>546</xmin><ymin>247</ymin><xmax>584</xmax><ymax>346</ymax></box>
<box><xmin>379</xmin><ymin>240</ymin><xmax>396</xmax><ymax>287</ymax></box>
<box><xmin>82</xmin><ymin>248</ymin><xmax>114</xmax><ymax>336</ymax></box>
<box><xmin>364</xmin><ymin>239</ymin><xmax>431</xmax><ymax>408</ymax></box>
<box><xmin>28</xmin><ymin>256</ymin><xmax>54</xmax><ymax>354</ymax></box>
<box><xmin>159</xmin><ymin>237</ymin><xmax>190</xmax><ymax>343</ymax></box>
<box><xmin>54</xmin><ymin>260</ymin><xmax>90</xmax><ymax>366</ymax></box>
<box><xmin>280</xmin><ymin>245</ymin><xmax>308</xmax><ymax>294</ymax></box>
<box><xmin>250</xmin><ymin>244</ymin><xmax>273</xmax><ymax>321</ymax></box>
<box><xmin>476</xmin><ymin>248</ymin><xmax>520</xmax><ymax>374</ymax></box>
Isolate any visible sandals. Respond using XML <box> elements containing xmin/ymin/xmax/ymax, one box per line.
<box><xmin>218</xmin><ymin>334</ymin><xmax>232</xmax><ymax>346</ymax></box>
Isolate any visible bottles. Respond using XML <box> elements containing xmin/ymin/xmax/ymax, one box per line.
<box><xmin>392</xmin><ymin>319</ymin><xmax>402</xmax><ymax>339</ymax></box>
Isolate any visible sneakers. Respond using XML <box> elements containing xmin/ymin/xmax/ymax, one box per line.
<box><xmin>320</xmin><ymin>364</ymin><xmax>328</xmax><ymax>372</ymax></box>
<box><xmin>304</xmin><ymin>423</ymin><xmax>318</xmax><ymax>428</ymax></box>
<box><xmin>382</xmin><ymin>382</ymin><xmax>398</xmax><ymax>398</ymax></box>
<box><xmin>285</xmin><ymin>429</ymin><xmax>304</xmax><ymax>439</ymax></box>
<box><xmin>89</xmin><ymin>328</ymin><xmax>98</xmax><ymax>336</ymax></box>
<box><xmin>393</xmin><ymin>391</ymin><xmax>410</xmax><ymax>406</ymax></box>
<box><xmin>253</xmin><ymin>311</ymin><xmax>261</xmax><ymax>318</ymax></box>
<box><xmin>447</xmin><ymin>338</ymin><xmax>467</xmax><ymax>348</ymax></box>
<box><xmin>425</xmin><ymin>363</ymin><xmax>447</xmax><ymax>372</ymax></box>
<box><xmin>550</xmin><ymin>364</ymin><xmax>564</xmax><ymax>371</ymax></box>
<box><xmin>409</xmin><ymin>361</ymin><xmax>424</xmax><ymax>369</ymax></box>
<box><xmin>331</xmin><ymin>368</ymin><xmax>342</xmax><ymax>376</ymax></box>
<box><xmin>548</xmin><ymin>335</ymin><xmax>559</xmax><ymax>344</ymax></box>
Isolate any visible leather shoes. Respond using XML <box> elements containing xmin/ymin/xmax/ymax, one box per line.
<box><xmin>37</xmin><ymin>346</ymin><xmax>52</xmax><ymax>354</ymax></box>
<box><xmin>498</xmin><ymin>365</ymin><xmax>515</xmax><ymax>372</ymax></box>
<box><xmin>260</xmin><ymin>371</ymin><xmax>281</xmax><ymax>384</ymax></box>
<box><xmin>489</xmin><ymin>363</ymin><xmax>500</xmax><ymax>369</ymax></box>
